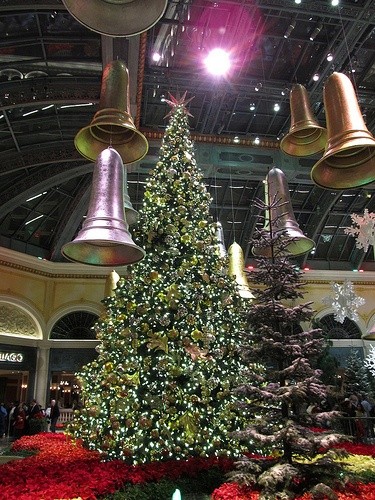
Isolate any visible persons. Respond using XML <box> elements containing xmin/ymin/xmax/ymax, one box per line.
<box><xmin>49</xmin><ymin>400</ymin><xmax>60</xmax><ymax>433</ymax></box>
<box><xmin>0</xmin><ymin>399</ymin><xmax>44</xmax><ymax>443</ymax></box>
